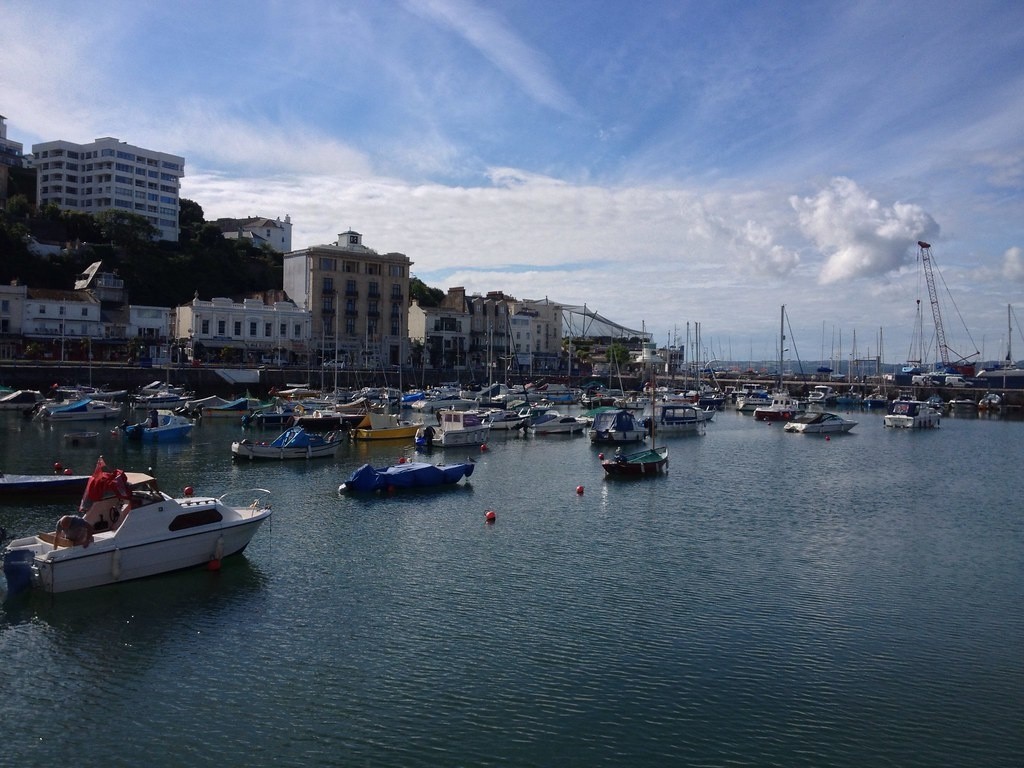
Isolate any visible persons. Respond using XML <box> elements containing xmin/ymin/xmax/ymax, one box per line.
<box><xmin>436</xmin><ymin>412</ymin><xmax>442</xmax><ymax>426</ymax></box>
<box><xmin>148</xmin><ymin>409</ymin><xmax>158</xmax><ymax>428</ymax></box>
<box><xmin>33</xmin><ymin>403</ymin><xmax>40</xmax><ymax>413</ymax></box>
<box><xmin>147</xmin><ymin>470</ymin><xmax>161</xmax><ymax>494</ymax></box>
<box><xmin>119</xmin><ymin>416</ymin><xmax>127</xmax><ymax>431</ymax></box>
<box><xmin>53</xmin><ymin>515</ymin><xmax>94</xmax><ymax>550</ymax></box>
<box><xmin>616</xmin><ymin>447</ymin><xmax>627</xmax><ymax>461</ymax></box>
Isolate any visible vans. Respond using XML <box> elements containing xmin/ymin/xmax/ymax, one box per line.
<box><xmin>944</xmin><ymin>376</ymin><xmax>974</xmax><ymax>388</ymax></box>
<box><xmin>261</xmin><ymin>354</ymin><xmax>288</xmax><ymax>364</ymax></box>
<box><xmin>910</xmin><ymin>375</ymin><xmax>940</xmax><ymax>387</ymax></box>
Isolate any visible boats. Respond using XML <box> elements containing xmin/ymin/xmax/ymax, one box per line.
<box><xmin>64</xmin><ymin>430</ymin><xmax>100</xmax><ymax>445</ymax></box>
<box><xmin>26</xmin><ymin>389</ymin><xmax>127</xmax><ymax>423</ymax></box>
<box><xmin>0</xmin><ymin>389</ymin><xmax>58</xmax><ymax>411</ymax></box>
<box><xmin>231</xmin><ymin>426</ymin><xmax>345</xmax><ymax>461</ymax></box>
<box><xmin>112</xmin><ymin>409</ymin><xmax>196</xmax><ymax>444</ymax></box>
<box><xmin>337</xmin><ymin>456</ymin><xmax>475</xmax><ymax>494</ymax></box>
<box><xmin>3</xmin><ymin>454</ymin><xmax>275</xmax><ymax>597</ymax></box>
<box><xmin>0</xmin><ymin>470</ymin><xmax>92</xmax><ymax>493</ymax></box>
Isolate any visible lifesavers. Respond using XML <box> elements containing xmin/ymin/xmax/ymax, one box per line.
<box><xmin>294</xmin><ymin>405</ymin><xmax>305</xmax><ymax>416</ymax></box>
<box><xmin>785</xmin><ymin>413</ymin><xmax>789</xmax><ymax>418</ymax></box>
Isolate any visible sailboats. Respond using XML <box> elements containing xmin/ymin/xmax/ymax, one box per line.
<box><xmin>132</xmin><ymin>304</ymin><xmax>1024</xmax><ymax>474</ymax></box>
<box><xmin>55</xmin><ymin>336</ymin><xmax>111</xmax><ymax>396</ymax></box>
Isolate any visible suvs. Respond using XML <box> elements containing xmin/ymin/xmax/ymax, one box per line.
<box><xmin>321</xmin><ymin>359</ymin><xmax>345</xmax><ymax>369</ymax></box>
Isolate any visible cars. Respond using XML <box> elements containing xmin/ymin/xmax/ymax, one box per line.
<box><xmin>781</xmin><ymin>368</ymin><xmax>795</xmax><ymax>380</ymax></box>
